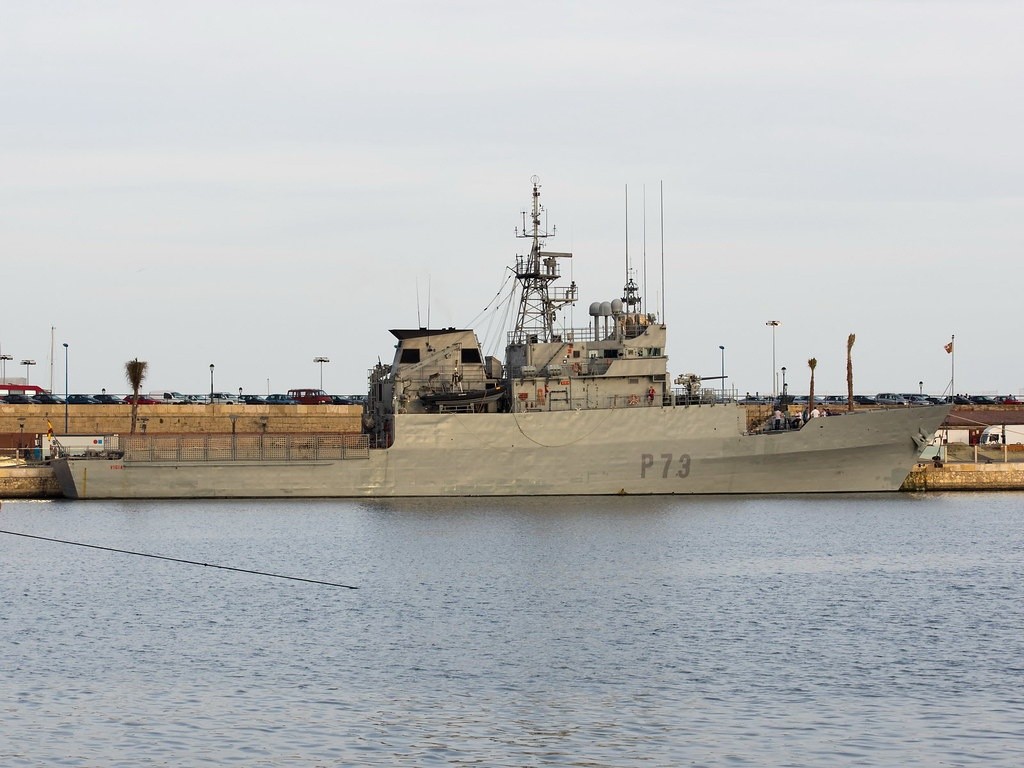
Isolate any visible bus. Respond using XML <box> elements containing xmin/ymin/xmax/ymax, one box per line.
<box><xmin>0</xmin><ymin>384</ymin><xmax>47</xmax><ymax>399</ymax></box>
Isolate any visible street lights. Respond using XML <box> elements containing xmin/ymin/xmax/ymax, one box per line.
<box><xmin>209</xmin><ymin>364</ymin><xmax>215</xmax><ymax>403</ymax></box>
<box><xmin>765</xmin><ymin>321</ymin><xmax>781</xmax><ymax>397</ymax></box>
<box><xmin>20</xmin><ymin>360</ymin><xmax>36</xmax><ymax>385</ymax></box>
<box><xmin>719</xmin><ymin>345</ymin><xmax>725</xmax><ymax>403</ymax></box>
<box><xmin>63</xmin><ymin>343</ymin><xmax>68</xmax><ymax>433</ymax></box>
<box><xmin>313</xmin><ymin>356</ymin><xmax>330</xmax><ymax>395</ymax></box>
<box><xmin>0</xmin><ymin>354</ymin><xmax>14</xmax><ymax>385</ymax></box>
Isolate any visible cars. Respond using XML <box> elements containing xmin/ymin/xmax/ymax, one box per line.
<box><xmin>123</xmin><ymin>394</ymin><xmax>162</xmax><ymax>404</ymax></box>
<box><xmin>32</xmin><ymin>394</ymin><xmax>66</xmax><ymax>404</ymax></box>
<box><xmin>92</xmin><ymin>394</ymin><xmax>129</xmax><ymax>405</ymax></box>
<box><xmin>265</xmin><ymin>394</ymin><xmax>301</xmax><ymax>405</ymax></box>
<box><xmin>4</xmin><ymin>394</ymin><xmax>43</xmax><ymax>404</ymax></box>
<box><xmin>739</xmin><ymin>391</ymin><xmax>1024</xmax><ymax>408</ymax></box>
<box><xmin>148</xmin><ymin>390</ymin><xmax>246</xmax><ymax>405</ymax></box>
<box><xmin>331</xmin><ymin>393</ymin><xmax>364</xmax><ymax>405</ymax></box>
<box><xmin>64</xmin><ymin>393</ymin><xmax>100</xmax><ymax>405</ymax></box>
<box><xmin>238</xmin><ymin>394</ymin><xmax>271</xmax><ymax>405</ymax></box>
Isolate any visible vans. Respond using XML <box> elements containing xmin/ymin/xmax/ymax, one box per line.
<box><xmin>287</xmin><ymin>389</ymin><xmax>334</xmax><ymax>405</ymax></box>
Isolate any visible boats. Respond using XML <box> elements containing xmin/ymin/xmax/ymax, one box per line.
<box><xmin>419</xmin><ymin>384</ymin><xmax>506</xmax><ymax>408</ymax></box>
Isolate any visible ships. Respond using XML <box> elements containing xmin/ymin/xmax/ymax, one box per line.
<box><xmin>44</xmin><ymin>170</ymin><xmax>956</xmax><ymax>504</ymax></box>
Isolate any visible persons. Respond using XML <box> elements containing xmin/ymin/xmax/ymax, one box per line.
<box><xmin>648</xmin><ymin>386</ymin><xmax>656</xmax><ymax>405</ymax></box>
<box><xmin>24</xmin><ymin>444</ymin><xmax>31</xmax><ymax>460</ymax></box>
<box><xmin>33</xmin><ymin>445</ymin><xmax>41</xmax><ymax>460</ymax></box>
<box><xmin>775</xmin><ymin>406</ymin><xmax>832</xmax><ymax>429</ymax></box>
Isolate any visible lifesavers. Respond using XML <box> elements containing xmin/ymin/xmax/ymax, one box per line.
<box><xmin>629</xmin><ymin>394</ymin><xmax>639</xmax><ymax>404</ymax></box>
